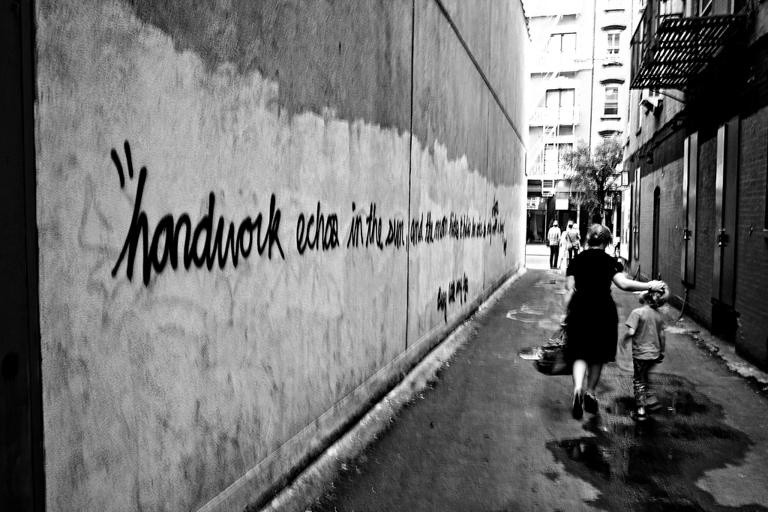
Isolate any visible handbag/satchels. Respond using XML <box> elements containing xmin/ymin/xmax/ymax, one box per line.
<box><xmin>533</xmin><ymin>322</ymin><xmax>574</xmax><ymax>376</ymax></box>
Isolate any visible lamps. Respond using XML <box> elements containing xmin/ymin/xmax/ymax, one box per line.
<box><xmin>640</xmin><ymin>96</ymin><xmax>664</xmax><ymax>113</ymax></box>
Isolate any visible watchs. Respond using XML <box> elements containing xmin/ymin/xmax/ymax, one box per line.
<box><xmin>647</xmin><ymin>281</ymin><xmax>653</xmax><ymax>292</ymax></box>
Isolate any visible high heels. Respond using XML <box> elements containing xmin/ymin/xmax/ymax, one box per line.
<box><xmin>582</xmin><ymin>391</ymin><xmax>600</xmax><ymax>414</ymax></box>
<box><xmin>570</xmin><ymin>388</ymin><xmax>584</xmax><ymax>420</ymax></box>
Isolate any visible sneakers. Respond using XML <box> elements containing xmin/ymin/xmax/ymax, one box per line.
<box><xmin>644</xmin><ymin>401</ymin><xmax>660</xmax><ymax>411</ymax></box>
<box><xmin>628</xmin><ymin>406</ymin><xmax>646</xmax><ymax>422</ymax></box>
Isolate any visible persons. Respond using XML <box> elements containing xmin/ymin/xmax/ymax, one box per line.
<box><xmin>547</xmin><ymin>220</ymin><xmax>562</xmax><ymax>268</ymax></box>
<box><xmin>619</xmin><ymin>279</ymin><xmax>668</xmax><ymax>421</ymax></box>
<box><xmin>564</xmin><ymin>223</ymin><xmax>666</xmax><ymax>420</ymax></box>
<box><xmin>565</xmin><ymin>222</ymin><xmax>581</xmax><ymax>265</ymax></box>
